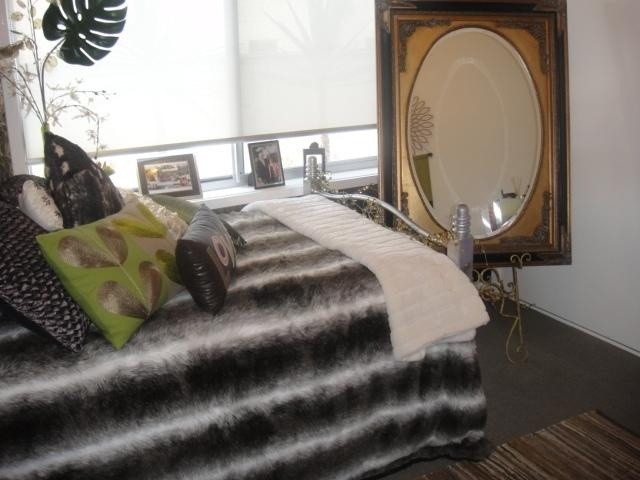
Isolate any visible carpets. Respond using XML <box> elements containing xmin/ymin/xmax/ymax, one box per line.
<box><xmin>411</xmin><ymin>405</ymin><xmax>638</xmax><ymax>479</ymax></box>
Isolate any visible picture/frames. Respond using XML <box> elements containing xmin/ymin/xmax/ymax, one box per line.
<box><xmin>248</xmin><ymin>138</ymin><xmax>288</xmax><ymax>190</ymax></box>
<box><xmin>136</xmin><ymin>150</ymin><xmax>204</xmax><ymax>203</ymax></box>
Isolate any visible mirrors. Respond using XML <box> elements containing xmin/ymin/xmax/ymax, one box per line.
<box><xmin>372</xmin><ymin>0</ymin><xmax>576</xmax><ymax>270</ymax></box>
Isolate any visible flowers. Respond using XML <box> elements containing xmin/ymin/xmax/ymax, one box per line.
<box><xmin>1</xmin><ymin>1</ymin><xmax>127</xmax><ymax>172</ymax></box>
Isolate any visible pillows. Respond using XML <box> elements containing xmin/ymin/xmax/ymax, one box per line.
<box><xmin>0</xmin><ymin>130</ymin><xmax>249</xmax><ymax>354</ymax></box>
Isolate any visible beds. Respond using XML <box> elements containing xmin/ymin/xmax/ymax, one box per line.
<box><xmin>0</xmin><ymin>153</ymin><xmax>500</xmax><ymax>480</ymax></box>
